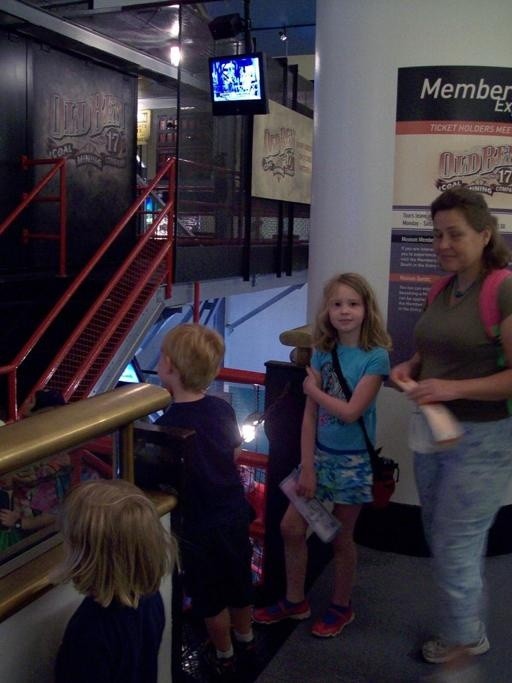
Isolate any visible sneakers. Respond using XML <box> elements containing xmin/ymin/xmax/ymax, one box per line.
<box><xmin>202</xmin><ymin>635</ymin><xmax>257</xmax><ymax>675</ymax></box>
<box><xmin>249</xmin><ymin>598</ymin><xmax>311</xmax><ymax>625</ymax></box>
<box><xmin>421</xmin><ymin>635</ymin><xmax>490</xmax><ymax>664</ymax></box>
<box><xmin>311</xmin><ymin>600</ymin><xmax>356</xmax><ymax>637</ymax></box>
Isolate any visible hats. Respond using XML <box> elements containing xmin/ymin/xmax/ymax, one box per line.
<box><xmin>30</xmin><ymin>389</ymin><xmax>69</xmax><ymax>412</ymax></box>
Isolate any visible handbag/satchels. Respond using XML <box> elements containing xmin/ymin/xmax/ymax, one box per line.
<box><xmin>370</xmin><ymin>457</ymin><xmax>398</xmax><ymax>508</ymax></box>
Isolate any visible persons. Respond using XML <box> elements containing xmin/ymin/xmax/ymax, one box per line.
<box><xmin>53</xmin><ymin>478</ymin><xmax>166</xmax><ymax>683</ymax></box>
<box><xmin>134</xmin><ymin>324</ymin><xmax>256</xmax><ymax>677</ymax></box>
<box><xmin>252</xmin><ymin>272</ymin><xmax>390</xmax><ymax>639</ymax></box>
<box><xmin>390</xmin><ymin>186</ymin><xmax>512</xmax><ymax>664</ymax></box>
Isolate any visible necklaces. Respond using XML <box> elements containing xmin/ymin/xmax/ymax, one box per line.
<box><xmin>454</xmin><ymin>276</ymin><xmax>473</xmax><ymax>297</ymax></box>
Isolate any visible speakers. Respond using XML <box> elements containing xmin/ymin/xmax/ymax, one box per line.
<box><xmin>208</xmin><ymin>12</ymin><xmax>242</xmax><ymax>40</ymax></box>
<box><xmin>209</xmin><ymin>51</ymin><xmax>269</xmax><ymax>116</ymax></box>
